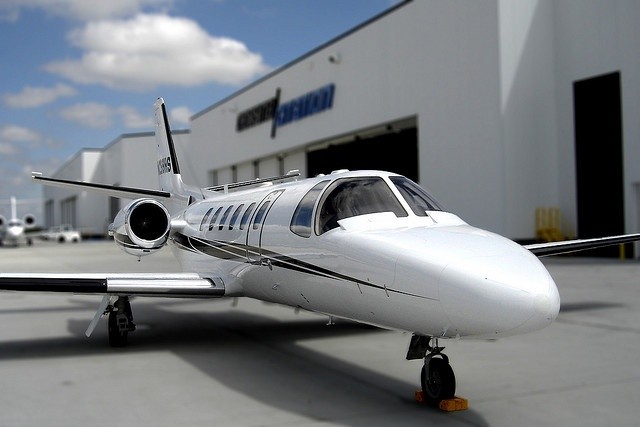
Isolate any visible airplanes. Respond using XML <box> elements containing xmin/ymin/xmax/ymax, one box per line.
<box><xmin>0</xmin><ymin>98</ymin><xmax>640</xmax><ymax>411</ymax></box>
<box><xmin>0</xmin><ymin>196</ymin><xmax>36</xmax><ymax>246</ymax></box>
